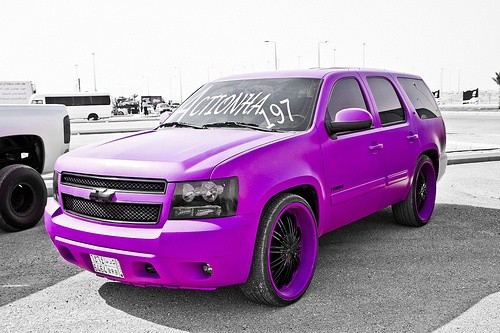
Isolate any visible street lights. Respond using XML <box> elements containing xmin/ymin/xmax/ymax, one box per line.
<box><xmin>264</xmin><ymin>41</ymin><xmax>277</xmax><ymax>71</ymax></box>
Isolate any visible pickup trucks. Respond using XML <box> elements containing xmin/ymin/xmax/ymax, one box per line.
<box><xmin>0</xmin><ymin>104</ymin><xmax>70</xmax><ymax>232</ymax></box>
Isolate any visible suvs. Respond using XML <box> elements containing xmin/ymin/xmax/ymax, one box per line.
<box><xmin>43</xmin><ymin>67</ymin><xmax>448</xmax><ymax>307</ymax></box>
<box><xmin>156</xmin><ymin>102</ymin><xmax>180</xmax><ymax>115</ymax></box>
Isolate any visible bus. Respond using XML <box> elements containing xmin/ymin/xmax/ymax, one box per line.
<box><xmin>29</xmin><ymin>94</ymin><xmax>113</xmax><ymax>120</ymax></box>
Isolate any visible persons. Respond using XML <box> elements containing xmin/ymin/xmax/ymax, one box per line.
<box><xmin>141</xmin><ymin>100</ymin><xmax>149</xmax><ymax>115</ymax></box>
